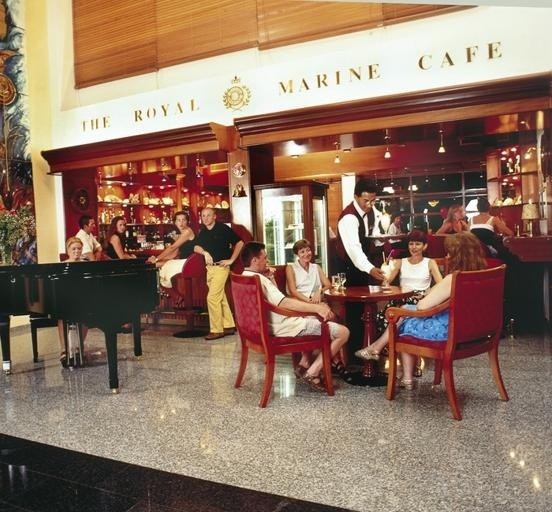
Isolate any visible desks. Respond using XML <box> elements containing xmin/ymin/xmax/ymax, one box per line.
<box><xmin>325</xmin><ymin>283</ymin><xmax>415</xmax><ymax>389</ymax></box>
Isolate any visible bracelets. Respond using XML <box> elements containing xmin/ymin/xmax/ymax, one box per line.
<box><xmin>201</xmin><ymin>249</ymin><xmax>204</xmax><ymax>255</ymax></box>
<box><xmin>156</xmin><ymin>257</ymin><xmax>159</xmax><ymax>262</ymax></box>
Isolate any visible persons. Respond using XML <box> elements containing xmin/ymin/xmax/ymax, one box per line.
<box><xmin>435</xmin><ymin>204</ymin><xmax>468</xmax><ymax>237</ymax></box>
<box><xmin>334</xmin><ymin>177</ymin><xmax>388</xmax><ymax>365</ymax></box>
<box><xmin>240</xmin><ymin>241</ymin><xmax>350</xmax><ymax>392</ymax></box>
<box><xmin>285</xmin><ymin>239</ymin><xmax>353</xmax><ymax>383</ymax></box>
<box><xmin>468</xmin><ymin>198</ymin><xmax>513</xmax><ymax>265</ymax></box>
<box><xmin>382</xmin><ymin>229</ymin><xmax>443</xmax><ymax>378</ymax></box>
<box><xmin>355</xmin><ymin>231</ymin><xmax>485</xmax><ymax>391</ymax></box>
<box><xmin>386</xmin><ymin>210</ymin><xmax>409</xmax><ymax>258</ymax></box>
<box><xmin>57</xmin><ymin>237</ymin><xmax>89</xmax><ymax>368</ymax></box>
<box><xmin>106</xmin><ymin>216</ymin><xmax>145</xmax><ymax>333</ymax></box>
<box><xmin>148</xmin><ymin>211</ymin><xmax>196</xmax><ymax>311</ymax></box>
<box><xmin>74</xmin><ymin>214</ymin><xmax>102</xmax><ymax>262</ymax></box>
<box><xmin>191</xmin><ymin>207</ymin><xmax>244</xmax><ymax>340</ymax></box>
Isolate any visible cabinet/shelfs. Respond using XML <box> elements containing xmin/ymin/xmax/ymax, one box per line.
<box><xmin>96</xmin><ymin>181</ymin><xmax>231</xmax><ymax>244</ymax></box>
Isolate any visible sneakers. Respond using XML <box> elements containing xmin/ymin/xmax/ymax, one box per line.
<box><xmin>60</xmin><ymin>351</ymin><xmax>71</xmax><ymax>366</ymax></box>
<box><xmin>73</xmin><ymin>345</ymin><xmax>84</xmax><ymax>364</ymax></box>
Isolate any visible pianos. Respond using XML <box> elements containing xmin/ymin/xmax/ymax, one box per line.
<box><xmin>2</xmin><ymin>262</ymin><xmax>159</xmax><ymax>396</ymax></box>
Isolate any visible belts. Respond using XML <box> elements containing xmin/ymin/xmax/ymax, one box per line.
<box><xmin>204</xmin><ymin>263</ymin><xmax>223</xmax><ymax>267</ymax></box>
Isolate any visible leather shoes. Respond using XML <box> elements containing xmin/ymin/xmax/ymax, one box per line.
<box><xmin>224</xmin><ymin>329</ymin><xmax>234</xmax><ymax>335</ymax></box>
<box><xmin>205</xmin><ymin>332</ymin><xmax>224</xmax><ymax>340</ymax></box>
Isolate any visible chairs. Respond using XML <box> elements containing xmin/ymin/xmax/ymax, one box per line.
<box><xmin>385</xmin><ymin>264</ymin><xmax>509</xmax><ymax>420</ymax></box>
<box><xmin>173</xmin><ymin>254</ymin><xmax>211</xmax><ymax>339</ymax></box>
<box><xmin>268</xmin><ymin>265</ymin><xmax>343</xmax><ymax>379</ymax></box>
<box><xmin>228</xmin><ymin>271</ymin><xmax>334</xmax><ymax>409</ymax></box>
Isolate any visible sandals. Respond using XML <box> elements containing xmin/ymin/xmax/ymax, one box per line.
<box><xmin>354</xmin><ymin>347</ymin><xmax>380</xmax><ymax>360</ymax></box>
<box><xmin>296</xmin><ymin>364</ymin><xmax>308</xmax><ymax>376</ymax></box>
<box><xmin>332</xmin><ymin>361</ymin><xmax>350</xmax><ymax>374</ymax></box>
<box><xmin>303</xmin><ymin>370</ymin><xmax>327</xmax><ymax>392</ymax></box>
<box><xmin>396</xmin><ymin>376</ymin><xmax>414</xmax><ymax>390</ymax></box>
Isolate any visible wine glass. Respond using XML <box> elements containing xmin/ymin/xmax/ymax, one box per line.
<box><xmin>338</xmin><ymin>272</ymin><xmax>346</xmax><ymax>290</ymax></box>
<box><xmin>331</xmin><ymin>276</ymin><xmax>341</xmax><ymax>295</ymax></box>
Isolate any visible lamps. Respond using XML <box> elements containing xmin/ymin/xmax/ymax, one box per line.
<box><xmin>521</xmin><ymin>203</ymin><xmax>540</xmax><ymax>238</ymax></box>
<box><xmin>128</xmin><ymin>162</ymin><xmax>134</xmax><ymax>186</ymax></box>
<box><xmin>383</xmin><ymin>129</ymin><xmax>392</xmax><ymax>159</ymax></box>
<box><xmin>334</xmin><ymin>142</ymin><xmax>340</xmax><ymax>164</ymax></box>
<box><xmin>162</xmin><ymin>161</ymin><xmax>168</xmax><ymax>183</ymax></box>
<box><xmin>98</xmin><ymin>170</ymin><xmax>103</xmax><ymax>189</ymax></box>
<box><xmin>196</xmin><ymin>154</ymin><xmax>202</xmax><ymax>178</ymax></box>
<box><xmin>436</xmin><ymin>127</ymin><xmax>447</xmax><ymax>154</ymax></box>
